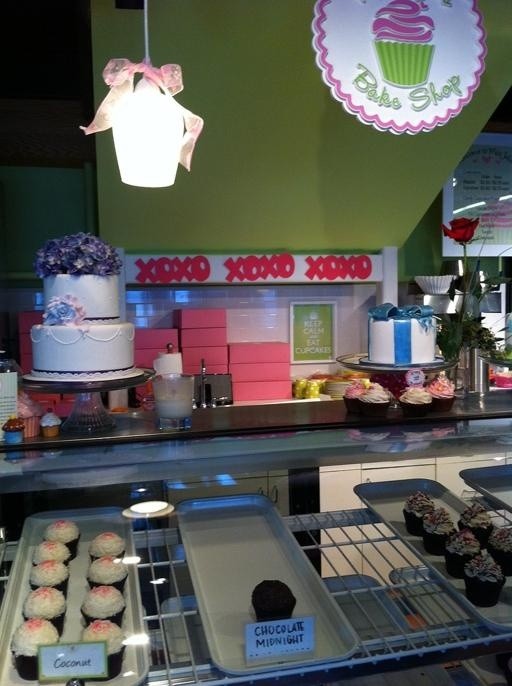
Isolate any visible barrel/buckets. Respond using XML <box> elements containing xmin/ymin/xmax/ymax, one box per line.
<box><xmin>151</xmin><ymin>373</ymin><xmax>194</xmax><ymax>418</ymax></box>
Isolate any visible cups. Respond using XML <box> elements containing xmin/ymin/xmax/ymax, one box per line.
<box><xmin>152</xmin><ymin>375</ymin><xmax>195</xmax><ymax>430</ymax></box>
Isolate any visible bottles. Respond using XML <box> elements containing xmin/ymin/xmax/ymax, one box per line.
<box><xmin>304</xmin><ymin>382</ymin><xmax>320</xmax><ymax>399</ymax></box>
<box><xmin>294</xmin><ymin>380</ymin><xmax>307</xmax><ymax>400</ymax></box>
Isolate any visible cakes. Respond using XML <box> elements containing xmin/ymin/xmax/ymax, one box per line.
<box><xmin>404</xmin><ymin>490</ymin><xmax>512</xmax><ymax>607</ymax></box>
<box><xmin>18</xmin><ymin>389</ymin><xmax>43</xmax><ymax>439</ymax></box>
<box><xmin>11</xmin><ymin>520</ymin><xmax>128</xmax><ymax>682</ymax></box>
<box><xmin>2</xmin><ymin>416</ymin><xmax>26</xmax><ymax>445</ymax></box>
<box><xmin>39</xmin><ymin>407</ymin><xmax>63</xmax><ymax>437</ymax></box>
<box><xmin>372</xmin><ymin>0</ymin><xmax>435</xmax><ymax>89</ymax></box>
<box><xmin>343</xmin><ymin>374</ymin><xmax>456</xmax><ymax>419</ymax></box>
<box><xmin>30</xmin><ymin>232</ymin><xmax>135</xmax><ymax>379</ymax></box>
<box><xmin>251</xmin><ymin>580</ymin><xmax>297</xmax><ymax>622</ymax></box>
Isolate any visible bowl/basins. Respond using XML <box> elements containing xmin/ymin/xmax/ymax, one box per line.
<box><xmin>414</xmin><ymin>274</ymin><xmax>455</xmax><ymax>295</ymax></box>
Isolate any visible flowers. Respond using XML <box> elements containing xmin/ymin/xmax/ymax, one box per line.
<box><xmin>426</xmin><ymin>215</ymin><xmax>511</xmax><ymax>364</ymax></box>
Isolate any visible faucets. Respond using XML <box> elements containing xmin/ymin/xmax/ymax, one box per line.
<box><xmin>200</xmin><ymin>358</ymin><xmax>208</xmax><ymax>408</ymax></box>
